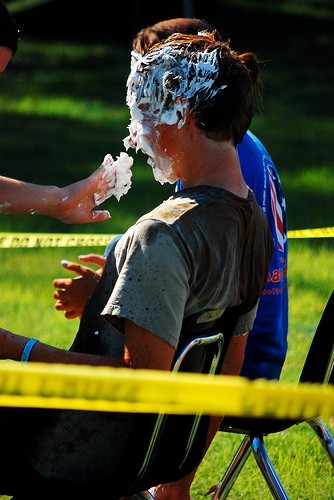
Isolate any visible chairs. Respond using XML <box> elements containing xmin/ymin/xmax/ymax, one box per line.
<box><xmin>122</xmin><ymin>286</ymin><xmax>262</xmax><ymax>500</ymax></box>
<box><xmin>213</xmin><ymin>292</ymin><xmax>334</xmax><ymax>500</ymax></box>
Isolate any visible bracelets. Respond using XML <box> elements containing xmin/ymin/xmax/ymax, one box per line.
<box><xmin>21</xmin><ymin>338</ymin><xmax>39</xmax><ymax>361</ymax></box>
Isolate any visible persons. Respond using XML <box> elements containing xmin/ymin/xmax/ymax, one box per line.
<box><xmin>0</xmin><ymin>2</ymin><xmax>135</xmax><ymax>225</ymax></box>
<box><xmin>52</xmin><ymin>18</ymin><xmax>289</xmax><ymax>382</ymax></box>
<box><xmin>0</xmin><ymin>31</ymin><xmax>276</xmax><ymax>500</ymax></box>
